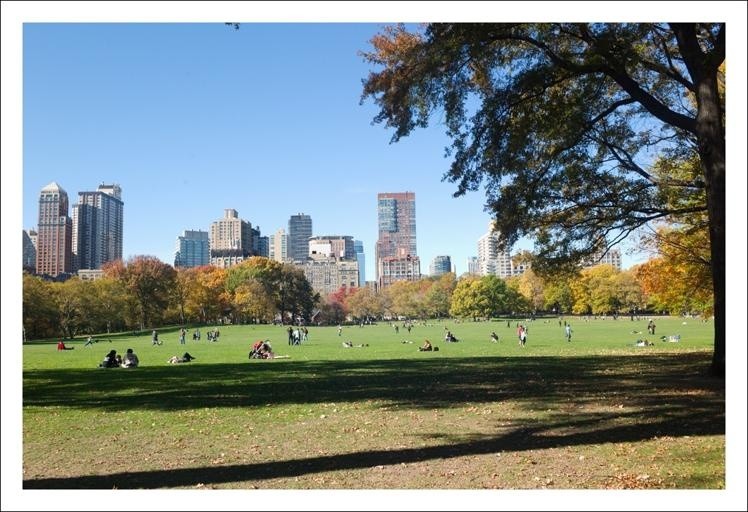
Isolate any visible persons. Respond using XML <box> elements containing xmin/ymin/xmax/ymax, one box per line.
<box><xmin>337</xmin><ymin>319</ymin><xmax>373</xmax><ymax>348</ymax></box>
<box><xmin>59</xmin><ymin>327</ymin><xmax>220</xmax><ymax>369</ymax></box>
<box><xmin>395</xmin><ymin>319</ymin><xmax>573</xmax><ymax>351</ymax></box>
<box><xmin>249</xmin><ymin>340</ymin><xmax>274</xmax><ymax>359</ymax></box>
<box><xmin>271</xmin><ymin>317</ymin><xmax>309</xmax><ymax>345</ymax></box>
<box><xmin>632</xmin><ymin>319</ymin><xmax>681</xmax><ymax>348</ymax></box>
